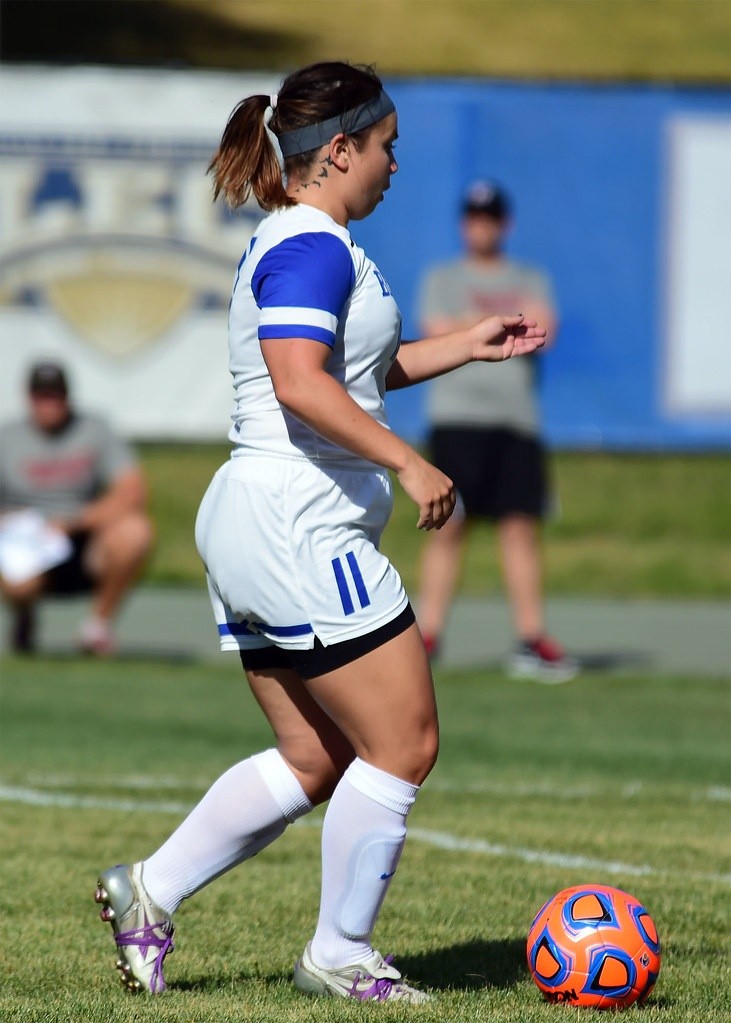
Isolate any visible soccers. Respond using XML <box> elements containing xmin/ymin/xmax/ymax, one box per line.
<box><xmin>524</xmin><ymin>883</ymin><xmax>661</xmax><ymax>1012</ymax></box>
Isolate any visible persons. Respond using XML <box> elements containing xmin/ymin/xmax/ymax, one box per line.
<box><xmin>2</xmin><ymin>355</ymin><xmax>162</xmax><ymax>664</ymax></box>
<box><xmin>406</xmin><ymin>170</ymin><xmax>591</xmax><ymax>686</ymax></box>
<box><xmin>92</xmin><ymin>56</ymin><xmax>554</xmax><ymax>1005</ymax></box>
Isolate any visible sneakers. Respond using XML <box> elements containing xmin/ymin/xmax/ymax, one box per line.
<box><xmin>76</xmin><ymin>617</ymin><xmax>120</xmax><ymax>659</ymax></box>
<box><xmin>290</xmin><ymin>939</ymin><xmax>437</xmax><ymax>1004</ymax></box>
<box><xmin>504</xmin><ymin>636</ymin><xmax>579</xmax><ymax>684</ymax></box>
<box><xmin>420</xmin><ymin>634</ymin><xmax>440</xmax><ymax>660</ymax></box>
<box><xmin>94</xmin><ymin>862</ymin><xmax>173</xmax><ymax>997</ymax></box>
